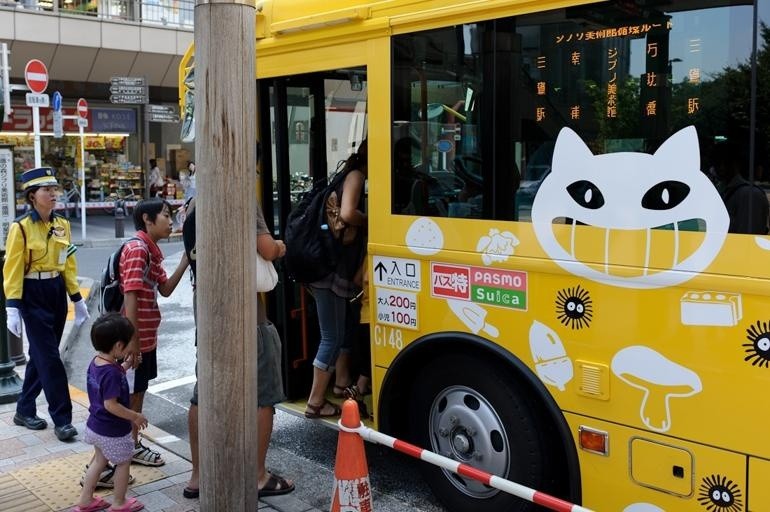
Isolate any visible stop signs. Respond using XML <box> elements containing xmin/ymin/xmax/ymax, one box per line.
<box><xmin>24</xmin><ymin>58</ymin><xmax>49</xmax><ymax>93</ymax></box>
<box><xmin>77</xmin><ymin>98</ymin><xmax>88</xmax><ymax>118</ymax></box>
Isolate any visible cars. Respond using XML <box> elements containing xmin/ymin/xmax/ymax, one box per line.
<box><xmin>517</xmin><ymin>163</ymin><xmax>550</xmax><ymax>199</ymax></box>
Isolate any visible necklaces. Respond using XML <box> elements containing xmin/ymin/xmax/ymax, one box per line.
<box><xmin>97</xmin><ymin>354</ymin><xmax>127</xmax><ymax>375</ymax></box>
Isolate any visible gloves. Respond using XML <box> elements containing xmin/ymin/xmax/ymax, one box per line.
<box><xmin>74</xmin><ymin>298</ymin><xmax>90</xmax><ymax>325</ymax></box>
<box><xmin>6</xmin><ymin>306</ymin><xmax>22</xmax><ymax>339</ymax></box>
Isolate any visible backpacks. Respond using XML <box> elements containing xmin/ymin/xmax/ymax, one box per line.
<box><xmin>98</xmin><ymin>237</ymin><xmax>152</xmax><ymax>318</ymax></box>
<box><xmin>280</xmin><ymin>166</ymin><xmax>365</xmax><ymax>283</ymax></box>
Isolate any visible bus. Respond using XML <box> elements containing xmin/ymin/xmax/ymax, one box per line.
<box><xmin>178</xmin><ymin>0</ymin><xmax>770</xmax><ymax>512</ymax></box>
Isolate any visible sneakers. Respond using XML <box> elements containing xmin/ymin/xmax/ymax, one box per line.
<box><xmin>344</xmin><ymin>385</ymin><xmax>369</xmax><ymax>417</ymax></box>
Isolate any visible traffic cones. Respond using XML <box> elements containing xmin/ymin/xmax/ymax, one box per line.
<box><xmin>328</xmin><ymin>398</ymin><xmax>374</xmax><ymax>511</ymax></box>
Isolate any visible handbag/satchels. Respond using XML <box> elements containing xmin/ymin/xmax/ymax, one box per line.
<box><xmin>258</xmin><ymin>248</ymin><xmax>279</xmax><ymax>293</ymax></box>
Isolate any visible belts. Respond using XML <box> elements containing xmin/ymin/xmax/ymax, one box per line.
<box><xmin>24</xmin><ymin>271</ymin><xmax>61</xmax><ymax>280</ymax></box>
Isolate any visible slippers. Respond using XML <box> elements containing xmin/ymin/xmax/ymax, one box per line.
<box><xmin>258</xmin><ymin>470</ymin><xmax>295</xmax><ymax>496</ymax></box>
<box><xmin>70</xmin><ymin>495</ymin><xmax>110</xmax><ymax>512</ymax></box>
<box><xmin>184</xmin><ymin>487</ymin><xmax>199</xmax><ymax>498</ymax></box>
<box><xmin>103</xmin><ymin>497</ymin><xmax>144</xmax><ymax>512</ymax></box>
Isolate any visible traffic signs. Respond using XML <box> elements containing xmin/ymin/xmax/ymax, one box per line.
<box><xmin>109</xmin><ymin>86</ymin><xmax>145</xmax><ymax>94</ymax></box>
<box><xmin>150</xmin><ymin>112</ymin><xmax>180</xmax><ymax>123</ymax></box>
<box><xmin>149</xmin><ymin>105</ymin><xmax>175</xmax><ymax>114</ymax></box>
<box><xmin>111</xmin><ymin>77</ymin><xmax>145</xmax><ymax>85</ymax></box>
<box><xmin>110</xmin><ymin>95</ymin><xmax>145</xmax><ymax>104</ymax></box>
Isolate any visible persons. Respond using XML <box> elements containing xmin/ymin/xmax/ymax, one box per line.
<box><xmin>186</xmin><ymin>159</ymin><xmax>196</xmax><ymax>196</ymax></box>
<box><xmin>1</xmin><ymin>164</ymin><xmax>94</xmax><ymax>442</ymax></box>
<box><xmin>76</xmin><ymin>311</ymin><xmax>150</xmax><ymax>512</ymax></box>
<box><xmin>179</xmin><ymin>141</ymin><xmax>297</xmax><ymax>500</ymax></box>
<box><xmin>145</xmin><ymin>157</ymin><xmax>168</xmax><ymax>197</ymax></box>
<box><xmin>76</xmin><ymin>197</ymin><xmax>190</xmax><ymax>493</ymax></box>
<box><xmin>283</xmin><ymin>133</ymin><xmax>375</xmax><ymax>424</ymax></box>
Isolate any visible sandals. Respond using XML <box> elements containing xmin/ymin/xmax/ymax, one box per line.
<box><xmin>131</xmin><ymin>441</ymin><xmax>165</xmax><ymax>466</ymax></box>
<box><xmin>333</xmin><ymin>383</ymin><xmax>369</xmax><ymax>397</ymax></box>
<box><xmin>305</xmin><ymin>398</ymin><xmax>341</xmax><ymax>419</ymax></box>
<box><xmin>80</xmin><ymin>465</ymin><xmax>135</xmax><ymax>488</ymax></box>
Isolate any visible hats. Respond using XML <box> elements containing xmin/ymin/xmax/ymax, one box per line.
<box><xmin>22</xmin><ymin>167</ymin><xmax>59</xmax><ymax>192</ymax></box>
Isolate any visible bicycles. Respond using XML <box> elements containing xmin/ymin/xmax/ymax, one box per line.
<box><xmin>57</xmin><ymin>178</ymin><xmax>93</xmax><ymax>215</ymax></box>
<box><xmin>272</xmin><ymin>175</ymin><xmax>311</xmax><ymax>205</ymax></box>
<box><xmin>103</xmin><ymin>186</ymin><xmax>138</xmax><ymax>214</ymax></box>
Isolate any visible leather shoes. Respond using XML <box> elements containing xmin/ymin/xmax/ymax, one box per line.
<box><xmin>54</xmin><ymin>424</ymin><xmax>77</xmax><ymax>441</ymax></box>
<box><xmin>14</xmin><ymin>411</ymin><xmax>47</xmax><ymax>430</ymax></box>
